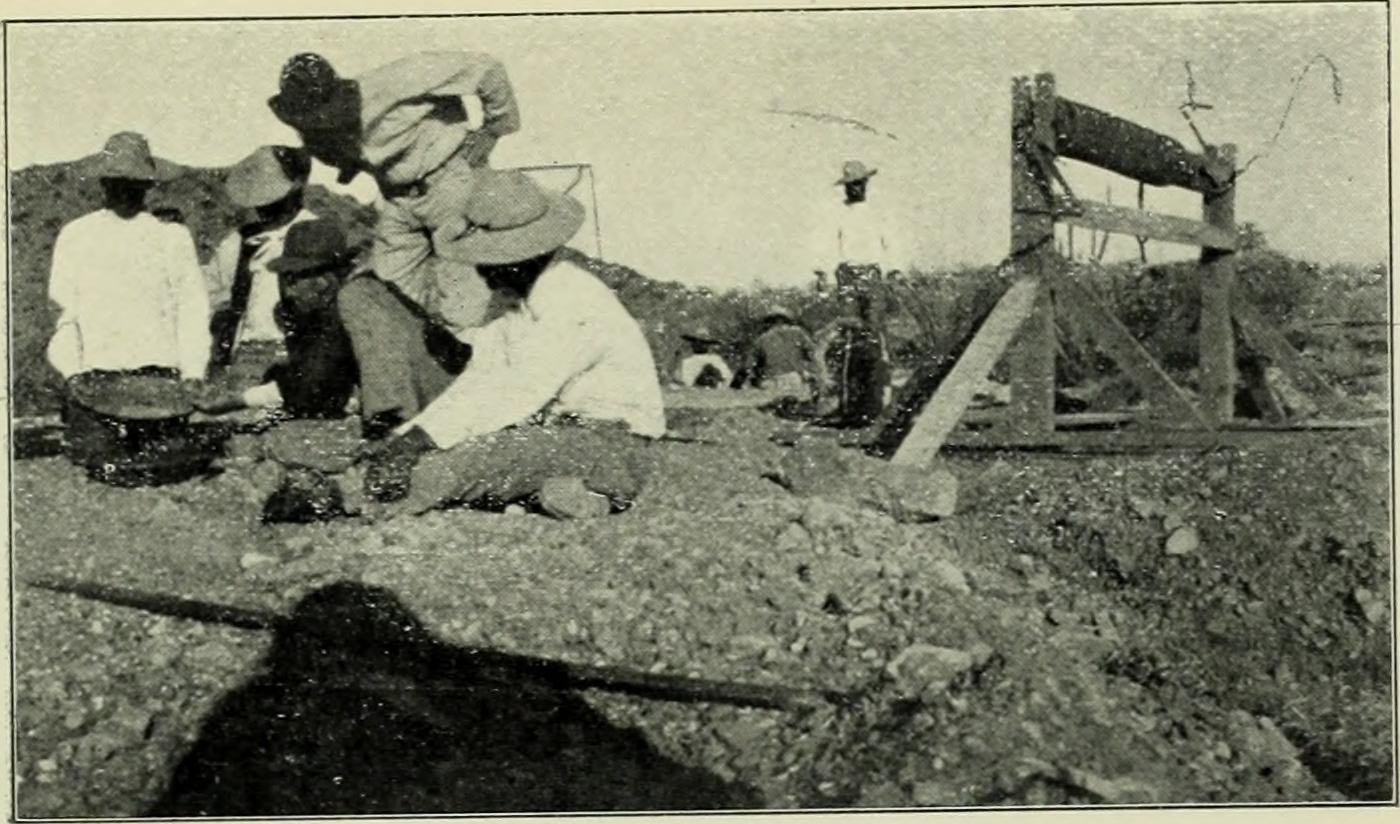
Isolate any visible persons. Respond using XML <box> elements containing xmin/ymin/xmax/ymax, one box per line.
<box><xmin>667</xmin><ymin>325</ymin><xmax>734</xmax><ymax>389</ymax></box>
<box><xmin>812</xmin><ymin>160</ymin><xmax>917</xmax><ymax>335</ymax></box>
<box><xmin>729</xmin><ymin>303</ymin><xmax>832</xmax><ymax>388</ymax></box>
<box><xmin>47</xmin><ymin>43</ymin><xmax>666</xmax><ymax>523</ymax></box>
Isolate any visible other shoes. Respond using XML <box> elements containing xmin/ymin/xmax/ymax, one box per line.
<box><xmin>540</xmin><ymin>474</ymin><xmax>609</xmax><ymax>517</ymax></box>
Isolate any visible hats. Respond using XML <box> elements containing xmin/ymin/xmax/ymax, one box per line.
<box><xmin>761</xmin><ymin>304</ymin><xmax>796</xmax><ymax>325</ymax></box>
<box><xmin>225</xmin><ymin>144</ymin><xmax>311</xmax><ymax>209</ymax></box>
<box><xmin>678</xmin><ymin>325</ymin><xmax>723</xmax><ymax>348</ymax></box>
<box><xmin>76</xmin><ymin>131</ymin><xmax>186</xmax><ymax>183</ymax></box>
<box><xmin>835</xmin><ymin>160</ymin><xmax>878</xmax><ymax>187</ymax></box>
<box><xmin>434</xmin><ymin>170</ymin><xmax>586</xmax><ymax>264</ymax></box>
<box><xmin>267</xmin><ymin>53</ymin><xmax>361</xmax><ymax>132</ymax></box>
<box><xmin>266</xmin><ymin>219</ymin><xmax>372</xmax><ymax>277</ymax></box>
